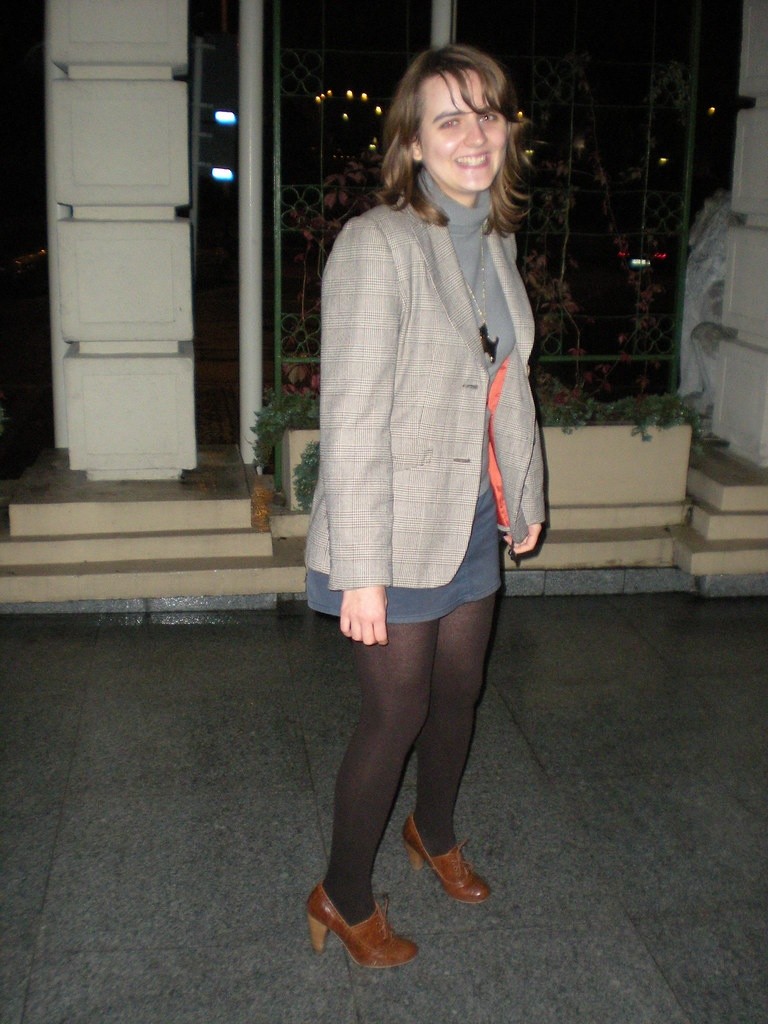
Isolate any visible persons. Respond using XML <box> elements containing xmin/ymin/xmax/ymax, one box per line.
<box><xmin>305</xmin><ymin>44</ymin><xmax>547</xmax><ymax>969</ymax></box>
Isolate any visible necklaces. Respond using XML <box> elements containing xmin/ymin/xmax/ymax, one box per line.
<box><xmin>440</xmin><ymin>225</ymin><xmax>501</xmax><ymax>367</ymax></box>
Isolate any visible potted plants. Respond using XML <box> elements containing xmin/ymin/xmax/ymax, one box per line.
<box><xmin>251</xmin><ymin>383</ymin><xmax>728</xmax><ymax>513</ymax></box>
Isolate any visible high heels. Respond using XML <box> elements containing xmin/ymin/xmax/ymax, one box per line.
<box><xmin>307</xmin><ymin>881</ymin><xmax>419</xmax><ymax>968</ymax></box>
<box><xmin>403</xmin><ymin>814</ymin><xmax>490</xmax><ymax>904</ymax></box>
